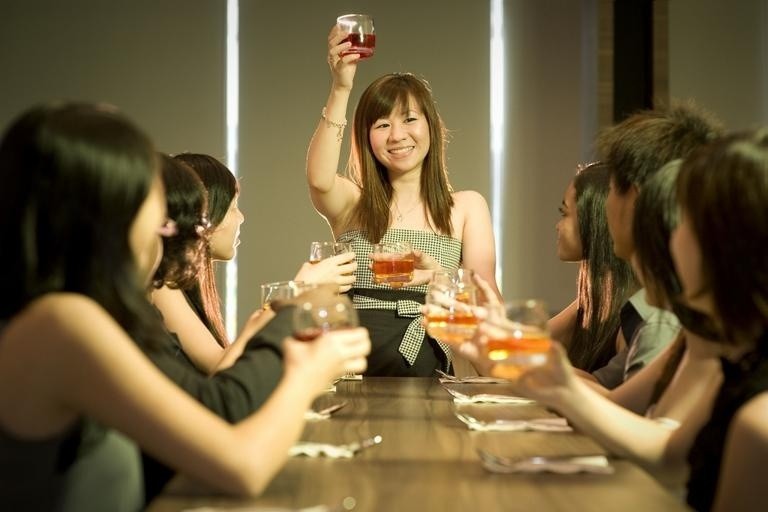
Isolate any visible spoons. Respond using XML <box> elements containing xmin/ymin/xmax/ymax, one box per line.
<box><xmin>320</xmin><ymin>402</ymin><xmax>348</xmax><ymax>416</ymax></box>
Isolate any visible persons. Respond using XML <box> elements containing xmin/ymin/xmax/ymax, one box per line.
<box><xmin>460</xmin><ymin>160</ymin><xmax>748</xmax><ymax>506</ymax></box>
<box><xmin>63</xmin><ymin>288</ymin><xmax>289</xmax><ymax>511</ymax></box>
<box><xmin>307</xmin><ymin>22</ymin><xmax>497</xmax><ymax>378</ymax></box>
<box><xmin>391</xmin><ymin>196</ymin><xmax>422</xmax><ymax>222</ymax></box>
<box><xmin>148</xmin><ymin>152</ymin><xmax>276</xmax><ymax>381</ymax></box>
<box><xmin>419</xmin><ymin>102</ymin><xmax>725</xmax><ymax>397</ymax></box>
<box><xmin>672</xmin><ymin>126</ymin><xmax>767</xmax><ymax>511</ymax></box>
<box><xmin>470</xmin><ymin>158</ymin><xmax>649</xmax><ymax>373</ymax></box>
<box><xmin>1</xmin><ymin>105</ymin><xmax>371</xmax><ymax>512</ymax></box>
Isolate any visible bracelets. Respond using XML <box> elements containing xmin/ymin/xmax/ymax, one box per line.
<box><xmin>320</xmin><ymin>107</ymin><xmax>349</xmax><ymax>142</ymax></box>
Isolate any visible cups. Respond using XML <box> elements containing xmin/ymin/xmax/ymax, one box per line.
<box><xmin>476</xmin><ymin>298</ymin><xmax>552</xmax><ymax>379</ymax></box>
<box><xmin>425</xmin><ymin>268</ymin><xmax>478</xmax><ymax>343</ymax></box>
<box><xmin>371</xmin><ymin>241</ymin><xmax>414</xmax><ymax>288</ymax></box>
<box><xmin>336</xmin><ymin>14</ymin><xmax>376</xmax><ymax>60</ymax></box>
<box><xmin>309</xmin><ymin>241</ymin><xmax>358</xmax><ymax>275</ymax></box>
<box><xmin>291</xmin><ymin>293</ymin><xmax>359</xmax><ymax>341</ymax></box>
<box><xmin>260</xmin><ymin>280</ymin><xmax>304</xmax><ymax>311</ymax></box>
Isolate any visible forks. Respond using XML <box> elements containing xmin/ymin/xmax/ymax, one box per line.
<box><xmin>435</xmin><ymin>368</ymin><xmax>611</xmax><ymax>474</ymax></box>
